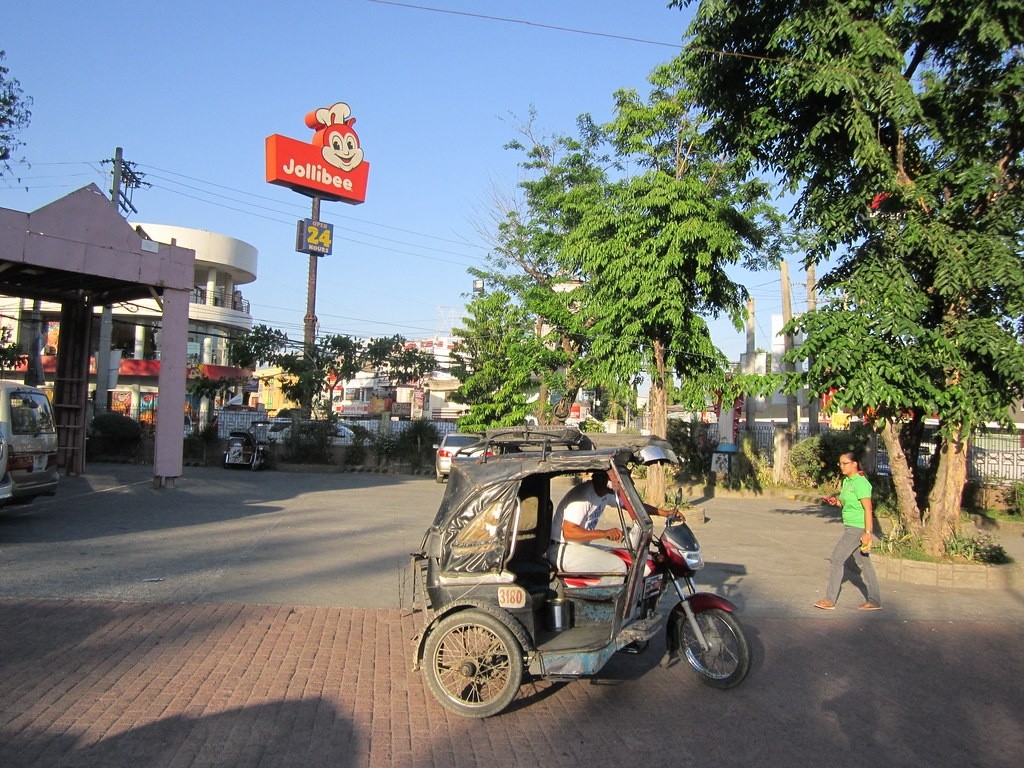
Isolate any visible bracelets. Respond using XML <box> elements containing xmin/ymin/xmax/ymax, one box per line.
<box><xmin>864</xmin><ymin>530</ymin><xmax>873</xmax><ymax>535</ymax></box>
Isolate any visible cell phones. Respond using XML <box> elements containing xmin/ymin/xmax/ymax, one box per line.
<box><xmin>821</xmin><ymin>497</ymin><xmax>835</xmax><ymax>503</ymax></box>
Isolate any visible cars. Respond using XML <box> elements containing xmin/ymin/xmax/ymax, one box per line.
<box><xmin>183</xmin><ymin>413</ymin><xmax>198</xmax><ymax>439</ymax></box>
<box><xmin>433</xmin><ymin>433</ymin><xmax>493</xmax><ymax>484</ymax></box>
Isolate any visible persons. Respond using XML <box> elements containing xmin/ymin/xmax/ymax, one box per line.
<box><xmin>546</xmin><ymin>470</ymin><xmax>687</xmax><ymax>586</ymax></box>
<box><xmin>814</xmin><ymin>450</ymin><xmax>883</xmax><ymax>611</ymax></box>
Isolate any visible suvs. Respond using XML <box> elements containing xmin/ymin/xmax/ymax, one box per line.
<box><xmin>0</xmin><ymin>379</ymin><xmax>61</xmax><ymax>509</ymax></box>
<box><xmin>266</xmin><ymin>419</ymin><xmax>372</xmax><ymax>447</ymax></box>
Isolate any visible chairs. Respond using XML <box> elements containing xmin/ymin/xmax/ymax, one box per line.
<box><xmin>531</xmin><ymin>496</ymin><xmax>622</xmax><ymax>600</ymax></box>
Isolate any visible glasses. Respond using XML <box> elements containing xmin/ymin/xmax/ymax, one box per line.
<box><xmin>837</xmin><ymin>462</ymin><xmax>855</xmax><ymax>466</ymax></box>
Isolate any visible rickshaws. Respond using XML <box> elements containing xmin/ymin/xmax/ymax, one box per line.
<box><xmin>399</xmin><ymin>429</ymin><xmax>754</xmax><ymax>720</ymax></box>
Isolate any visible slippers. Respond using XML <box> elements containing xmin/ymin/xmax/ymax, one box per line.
<box><xmin>814</xmin><ymin>599</ymin><xmax>836</xmax><ymax>610</ymax></box>
<box><xmin>857</xmin><ymin>603</ymin><xmax>882</xmax><ymax>610</ymax></box>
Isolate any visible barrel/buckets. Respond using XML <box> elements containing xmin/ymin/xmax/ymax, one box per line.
<box><xmin>544</xmin><ymin>598</ymin><xmax>570</xmax><ymax>631</ymax></box>
